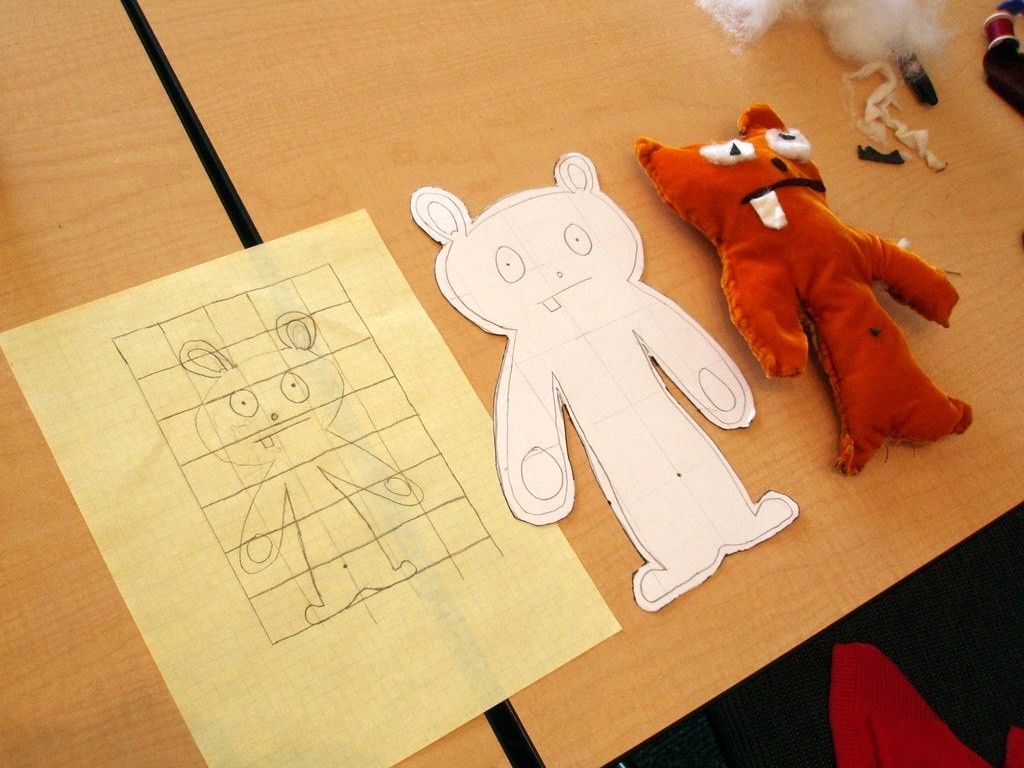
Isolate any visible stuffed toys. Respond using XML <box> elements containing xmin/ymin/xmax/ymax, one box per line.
<box><xmin>635</xmin><ymin>103</ymin><xmax>973</xmax><ymax>474</ymax></box>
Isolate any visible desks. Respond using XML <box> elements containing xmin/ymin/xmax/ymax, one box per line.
<box><xmin>139</xmin><ymin>0</ymin><xmax>1024</xmax><ymax>768</ymax></box>
<box><xmin>0</xmin><ymin>0</ymin><xmax>512</xmax><ymax>768</ymax></box>
